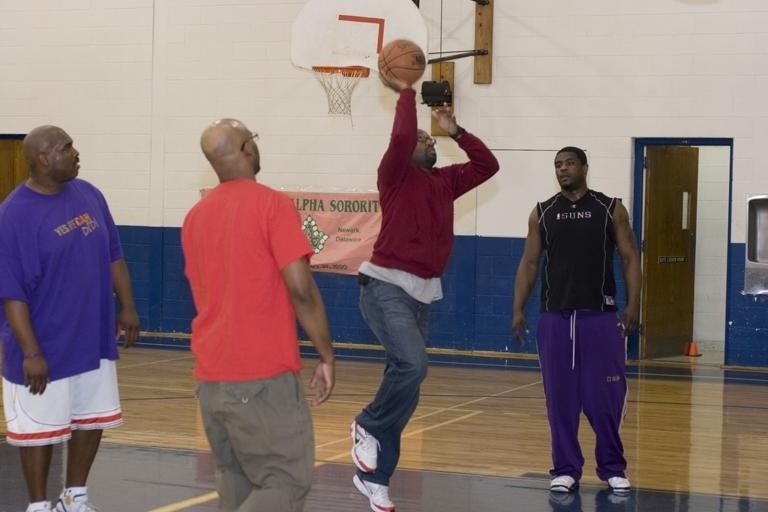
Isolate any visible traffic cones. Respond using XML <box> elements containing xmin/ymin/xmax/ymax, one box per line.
<box><xmin>685</xmin><ymin>340</ymin><xmax>701</xmax><ymax>356</ymax></box>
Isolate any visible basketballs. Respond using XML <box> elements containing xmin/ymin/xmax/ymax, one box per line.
<box><xmin>378</xmin><ymin>40</ymin><xmax>425</xmax><ymax>85</ymax></box>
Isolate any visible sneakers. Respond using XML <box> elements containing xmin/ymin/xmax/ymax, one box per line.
<box><xmin>605</xmin><ymin>475</ymin><xmax>632</xmax><ymax>494</ymax></box>
<box><xmin>54</xmin><ymin>485</ymin><xmax>103</xmax><ymax>512</ymax></box>
<box><xmin>548</xmin><ymin>475</ymin><xmax>580</xmax><ymax>494</ymax></box>
<box><xmin>352</xmin><ymin>471</ymin><xmax>397</xmax><ymax>512</ymax></box>
<box><xmin>349</xmin><ymin>418</ymin><xmax>381</xmax><ymax>475</ymax></box>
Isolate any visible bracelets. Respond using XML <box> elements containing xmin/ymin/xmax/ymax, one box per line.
<box><xmin>23</xmin><ymin>350</ymin><xmax>44</xmax><ymax>359</ymax></box>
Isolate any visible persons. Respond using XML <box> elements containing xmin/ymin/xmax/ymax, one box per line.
<box><xmin>347</xmin><ymin>67</ymin><xmax>501</xmax><ymax>512</ymax></box>
<box><xmin>512</xmin><ymin>146</ymin><xmax>644</xmax><ymax>494</ymax></box>
<box><xmin>0</xmin><ymin>125</ymin><xmax>141</xmax><ymax>512</ymax></box>
<box><xmin>181</xmin><ymin>118</ymin><xmax>336</xmax><ymax>512</ymax></box>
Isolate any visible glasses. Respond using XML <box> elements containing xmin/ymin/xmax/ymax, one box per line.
<box><xmin>239</xmin><ymin>130</ymin><xmax>260</xmax><ymax>152</ymax></box>
<box><xmin>415</xmin><ymin>131</ymin><xmax>438</xmax><ymax>145</ymax></box>
